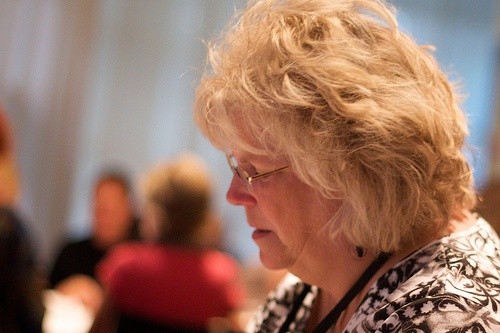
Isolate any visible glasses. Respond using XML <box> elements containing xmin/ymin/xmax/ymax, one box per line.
<box><xmin>225</xmin><ymin>151</ymin><xmax>292</xmax><ymax>192</ymax></box>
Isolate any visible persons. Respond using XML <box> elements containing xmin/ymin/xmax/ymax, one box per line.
<box><xmin>1</xmin><ymin>1</ymin><xmax>500</xmax><ymax>333</ymax></box>
